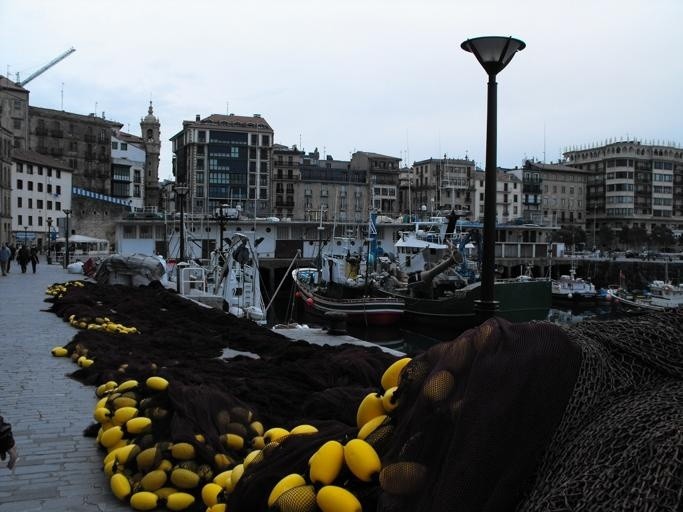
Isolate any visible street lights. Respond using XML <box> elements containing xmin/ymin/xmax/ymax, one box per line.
<box><xmin>46</xmin><ymin>215</ymin><xmax>53</xmax><ymax>265</ymax></box>
<box><xmin>458</xmin><ymin>35</ymin><xmax>526</xmax><ymax>324</ymax></box>
<box><xmin>61</xmin><ymin>208</ymin><xmax>73</xmax><ymax>270</ymax></box>
<box><xmin>23</xmin><ymin>226</ymin><xmax>28</xmax><ymax>246</ymax></box>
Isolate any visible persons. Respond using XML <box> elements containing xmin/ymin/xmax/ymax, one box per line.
<box><xmin>0</xmin><ymin>413</ymin><xmax>17</xmax><ymax>470</ymax></box>
<box><xmin>0</xmin><ymin>239</ymin><xmax>88</xmax><ymax>277</ymax></box>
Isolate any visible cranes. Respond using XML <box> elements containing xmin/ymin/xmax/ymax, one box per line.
<box><xmin>13</xmin><ymin>45</ymin><xmax>75</xmax><ymax>87</ymax></box>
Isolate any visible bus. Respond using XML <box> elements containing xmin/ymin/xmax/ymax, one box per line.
<box><xmin>51</xmin><ymin>235</ymin><xmax>110</xmax><ymax>265</ymax></box>
